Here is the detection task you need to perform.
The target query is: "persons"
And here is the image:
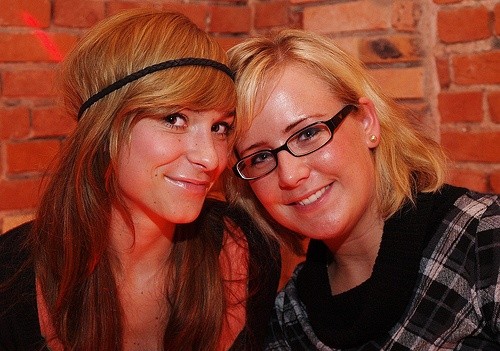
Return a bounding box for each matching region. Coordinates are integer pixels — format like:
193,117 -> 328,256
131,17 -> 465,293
0,8 -> 284,351
220,29 -> 500,351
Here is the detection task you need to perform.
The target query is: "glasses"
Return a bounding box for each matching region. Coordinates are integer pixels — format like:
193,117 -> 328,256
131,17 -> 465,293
232,104 -> 354,182
